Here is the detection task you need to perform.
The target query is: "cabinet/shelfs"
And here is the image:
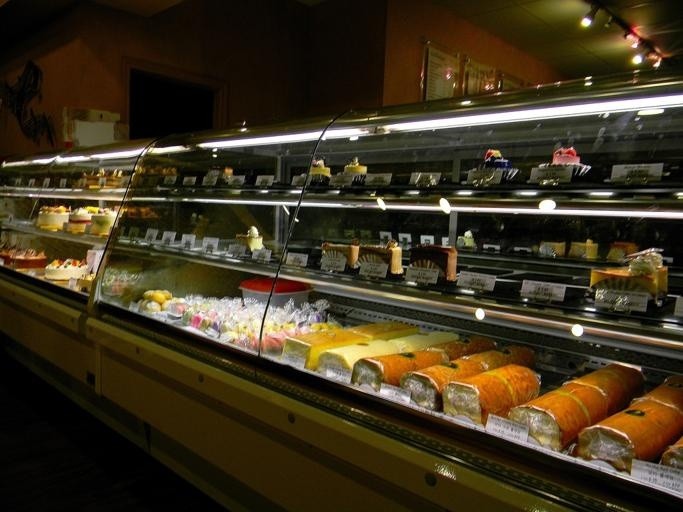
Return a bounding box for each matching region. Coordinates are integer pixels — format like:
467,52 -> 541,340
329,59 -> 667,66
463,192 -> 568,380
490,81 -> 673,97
0,139 -> 158,396
86,74 -> 683,511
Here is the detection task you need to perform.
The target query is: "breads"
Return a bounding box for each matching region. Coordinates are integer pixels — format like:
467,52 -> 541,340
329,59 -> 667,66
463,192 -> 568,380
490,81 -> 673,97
283,321 -> 418,371
137,288 -> 177,312
316,329 -> 461,380
399,345 -> 537,411
441,364 -> 542,426
660,436 -> 683,469
571,375 -> 681,472
507,363 -> 648,453
349,335 -> 498,392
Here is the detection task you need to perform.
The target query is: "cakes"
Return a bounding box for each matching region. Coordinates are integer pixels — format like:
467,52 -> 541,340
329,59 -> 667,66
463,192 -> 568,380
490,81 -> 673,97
357,239 -> 403,274
43,258 -> 88,280
35,206 -> 70,230
589,252 -> 668,295
5,247 -> 47,269
409,244 -> 457,281
568,239 -> 597,258
538,240 -> 566,257
309,158 -> 330,177
319,238 -> 360,268
343,157 -> 367,175
91,212 -> 116,234
62,206 -> 104,234
236,225 -> 265,251
551,146 -> 579,164
607,242 -> 632,259
74,169 -> 125,190
478,148 -> 510,169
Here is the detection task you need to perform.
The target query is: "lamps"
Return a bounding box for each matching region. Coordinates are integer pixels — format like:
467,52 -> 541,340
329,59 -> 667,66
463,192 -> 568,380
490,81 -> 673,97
577,3 -> 679,72
